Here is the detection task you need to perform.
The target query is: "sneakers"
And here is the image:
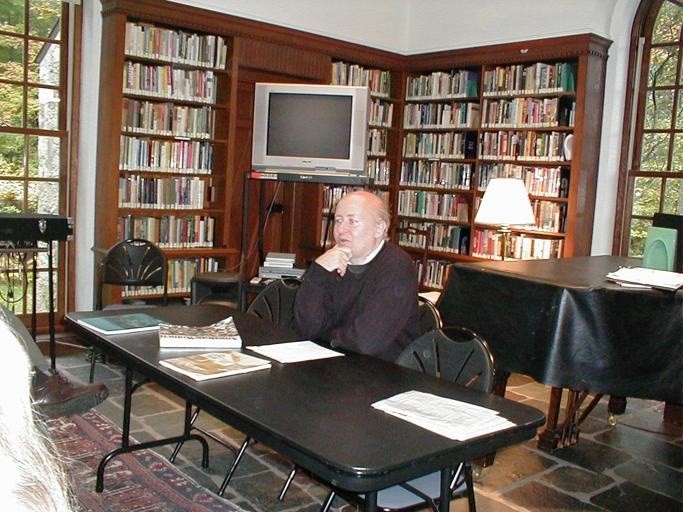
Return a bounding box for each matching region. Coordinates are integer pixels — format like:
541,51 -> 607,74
29,368 -> 109,420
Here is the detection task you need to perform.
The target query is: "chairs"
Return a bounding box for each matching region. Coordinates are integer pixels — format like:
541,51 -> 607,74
91,238 -> 168,383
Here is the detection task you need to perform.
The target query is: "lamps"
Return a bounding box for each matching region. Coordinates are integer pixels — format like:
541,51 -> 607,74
475,179 -> 538,261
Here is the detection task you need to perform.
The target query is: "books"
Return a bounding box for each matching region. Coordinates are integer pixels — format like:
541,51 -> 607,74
614,281 -> 652,288
77,313 -> 170,335
265,251 -> 297,264
158,347 -> 272,382
399,72 -> 478,288
321,51 -> 390,246
473,62 -> 576,259
605,265 -> 683,292
263,262 -> 294,268
158,315 -> 243,350
122,18 -> 228,298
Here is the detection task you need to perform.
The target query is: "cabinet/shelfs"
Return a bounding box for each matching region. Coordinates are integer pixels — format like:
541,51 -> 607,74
91,1 -> 612,363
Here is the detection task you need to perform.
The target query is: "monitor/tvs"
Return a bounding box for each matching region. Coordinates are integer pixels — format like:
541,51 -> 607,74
251,82 -> 371,176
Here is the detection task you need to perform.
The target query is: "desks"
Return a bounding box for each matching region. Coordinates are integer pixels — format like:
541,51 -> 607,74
450,254 -> 681,468
60,303 -> 545,512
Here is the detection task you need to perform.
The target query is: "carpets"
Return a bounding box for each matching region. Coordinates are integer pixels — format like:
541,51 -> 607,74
37,408 -> 251,512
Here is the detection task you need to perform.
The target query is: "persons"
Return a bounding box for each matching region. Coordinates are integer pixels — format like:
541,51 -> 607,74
0,303 -> 108,421
0,319 -> 77,512
290,189 -> 422,364
